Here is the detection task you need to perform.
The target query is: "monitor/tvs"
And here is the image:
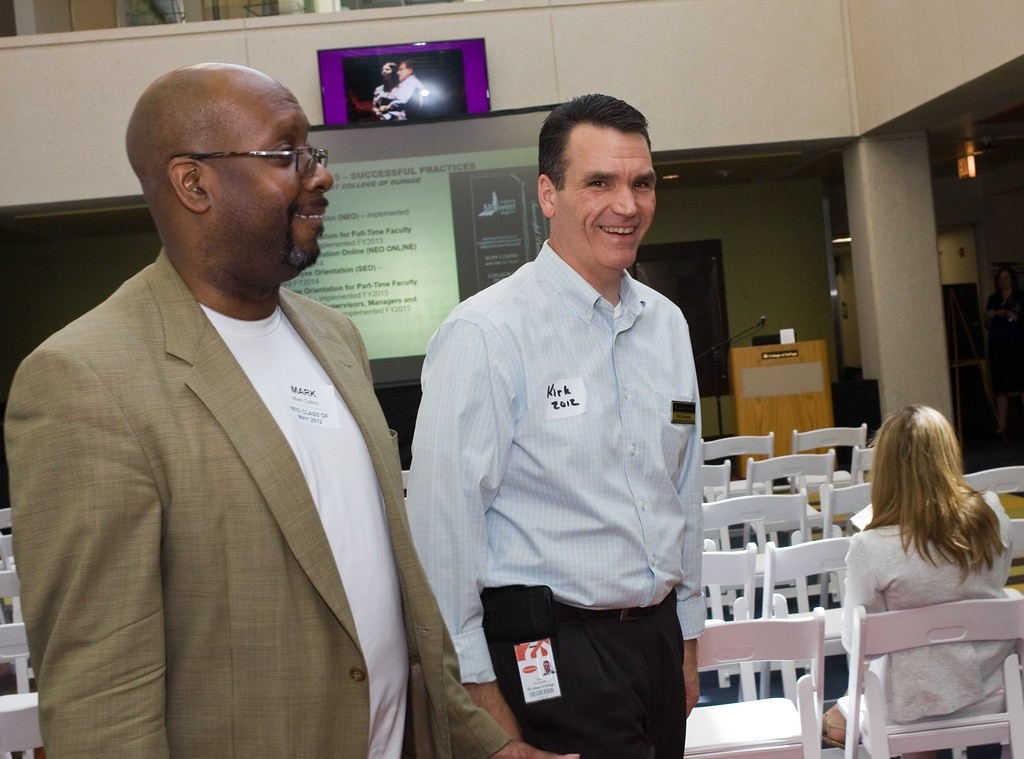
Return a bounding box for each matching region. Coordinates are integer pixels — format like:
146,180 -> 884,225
317,36 -> 491,126
751,333 -> 797,348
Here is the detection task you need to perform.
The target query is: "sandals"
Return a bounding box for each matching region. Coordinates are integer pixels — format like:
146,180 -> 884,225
821,712 -> 846,751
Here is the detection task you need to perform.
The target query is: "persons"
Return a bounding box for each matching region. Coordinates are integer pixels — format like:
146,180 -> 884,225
985,266 -> 1024,434
543,660 -> 555,676
4,63 -> 578,759
372,61 -> 425,120
408,95 -> 705,759
822,405 -> 1014,759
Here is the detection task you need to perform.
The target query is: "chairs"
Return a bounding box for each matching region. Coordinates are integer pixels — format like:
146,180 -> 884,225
0,421 -> 1024,759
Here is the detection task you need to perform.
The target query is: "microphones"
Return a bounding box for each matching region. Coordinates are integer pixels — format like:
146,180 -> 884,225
761,316 -> 767,327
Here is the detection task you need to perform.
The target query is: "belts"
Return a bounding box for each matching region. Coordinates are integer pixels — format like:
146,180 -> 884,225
555,587 -> 675,626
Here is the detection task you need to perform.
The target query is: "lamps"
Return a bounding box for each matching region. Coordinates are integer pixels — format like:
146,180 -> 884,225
957,154 -> 976,178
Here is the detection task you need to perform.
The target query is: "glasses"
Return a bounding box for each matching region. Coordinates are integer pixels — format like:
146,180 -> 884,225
170,146 -> 328,178
